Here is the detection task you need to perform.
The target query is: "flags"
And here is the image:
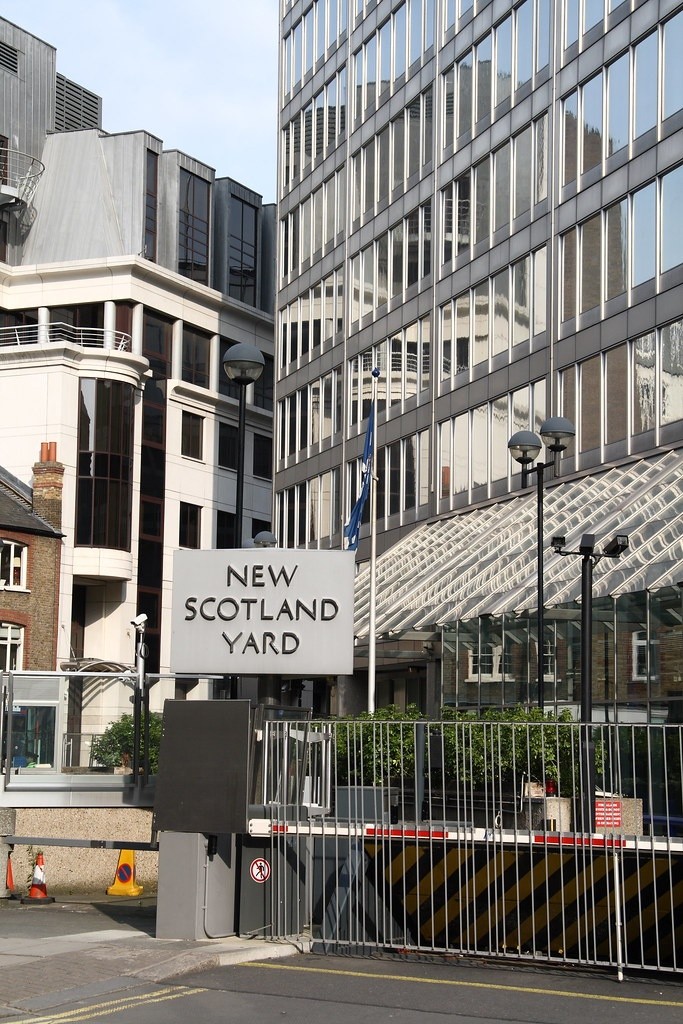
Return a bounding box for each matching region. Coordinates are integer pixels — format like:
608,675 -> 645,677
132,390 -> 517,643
343,381 -> 374,556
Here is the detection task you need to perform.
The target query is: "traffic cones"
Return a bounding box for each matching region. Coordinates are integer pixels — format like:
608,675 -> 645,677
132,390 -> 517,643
107,848 -> 144,897
21,851 -> 56,904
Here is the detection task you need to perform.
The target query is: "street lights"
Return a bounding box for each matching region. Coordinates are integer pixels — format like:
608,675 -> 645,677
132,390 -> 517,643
507,417 -> 576,720
224,344 -> 265,699
552,533 -> 629,834
